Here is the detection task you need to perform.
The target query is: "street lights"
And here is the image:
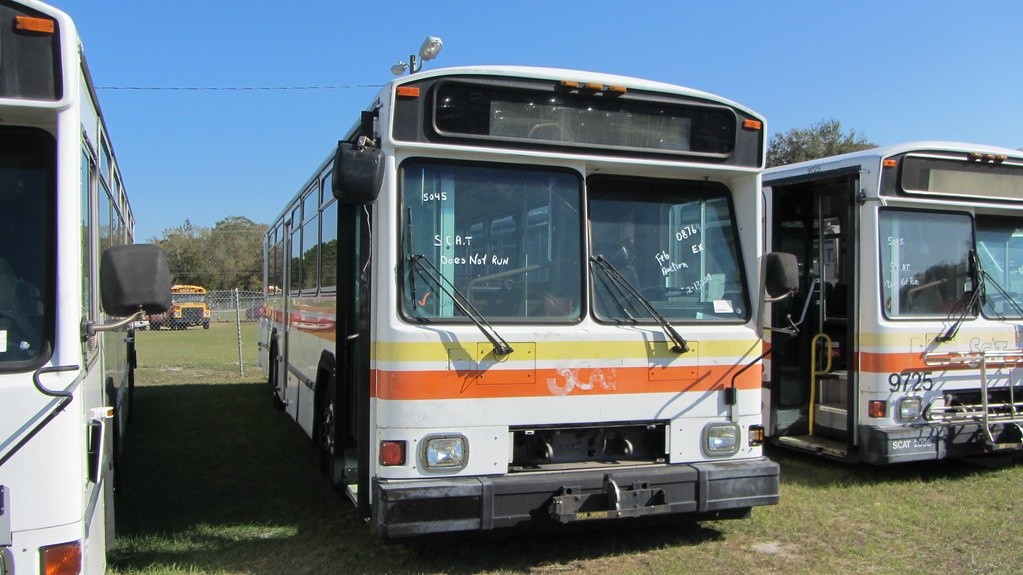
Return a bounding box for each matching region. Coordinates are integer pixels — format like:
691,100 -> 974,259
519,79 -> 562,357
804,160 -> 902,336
390,35 -> 443,79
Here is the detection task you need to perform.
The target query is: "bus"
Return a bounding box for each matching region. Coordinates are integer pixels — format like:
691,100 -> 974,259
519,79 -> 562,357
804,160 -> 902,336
148,285 -> 211,330
256,63 -> 800,547
0,0 -> 177,575
466,139 -> 1023,465
